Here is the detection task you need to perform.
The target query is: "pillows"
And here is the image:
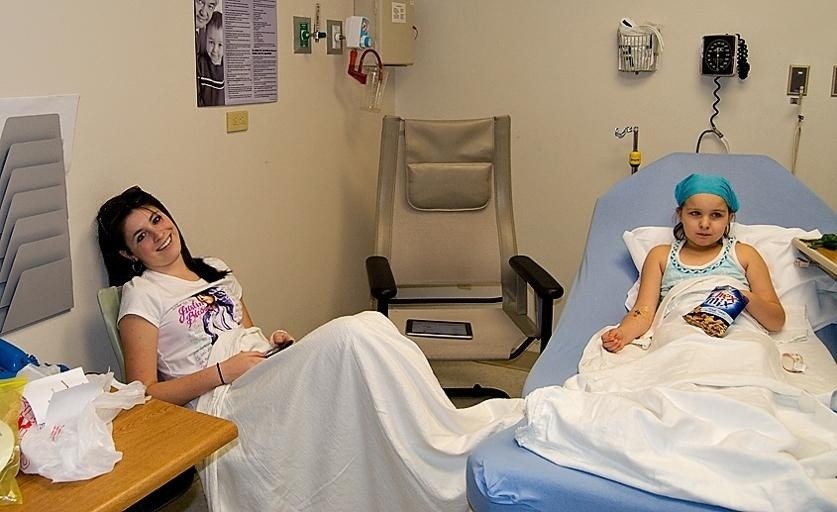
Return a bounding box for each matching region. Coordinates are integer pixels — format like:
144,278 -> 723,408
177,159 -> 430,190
621,217 -> 837,335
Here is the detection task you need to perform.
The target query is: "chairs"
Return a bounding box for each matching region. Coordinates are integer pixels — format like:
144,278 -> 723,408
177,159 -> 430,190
95,279 -> 138,384
361,110 -> 564,404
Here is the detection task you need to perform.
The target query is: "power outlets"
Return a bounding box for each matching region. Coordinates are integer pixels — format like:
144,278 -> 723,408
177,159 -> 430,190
224,108 -> 249,134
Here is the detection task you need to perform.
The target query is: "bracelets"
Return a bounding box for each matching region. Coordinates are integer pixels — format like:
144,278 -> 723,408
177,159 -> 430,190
214,360 -> 228,387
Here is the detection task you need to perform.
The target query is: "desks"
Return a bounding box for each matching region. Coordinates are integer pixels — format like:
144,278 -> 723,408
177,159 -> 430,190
0,386 -> 240,511
789,223 -> 835,279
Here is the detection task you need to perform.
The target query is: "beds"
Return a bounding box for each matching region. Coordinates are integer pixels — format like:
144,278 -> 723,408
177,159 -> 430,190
464,149 -> 836,509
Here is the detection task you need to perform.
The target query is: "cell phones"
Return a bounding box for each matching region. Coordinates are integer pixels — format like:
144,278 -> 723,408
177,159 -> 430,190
262,340 -> 293,358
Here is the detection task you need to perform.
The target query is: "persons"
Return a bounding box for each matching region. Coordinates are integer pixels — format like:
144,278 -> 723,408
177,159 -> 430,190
94,185 -> 296,408
196,11 -> 224,105
194,0 -> 218,55
600,174 -> 787,352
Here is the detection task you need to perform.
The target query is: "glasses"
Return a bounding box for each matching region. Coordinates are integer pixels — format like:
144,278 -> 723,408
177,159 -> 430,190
96,185 -> 141,229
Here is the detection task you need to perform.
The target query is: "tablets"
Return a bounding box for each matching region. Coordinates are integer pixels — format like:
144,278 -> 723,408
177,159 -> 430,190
406,318 -> 473,340
